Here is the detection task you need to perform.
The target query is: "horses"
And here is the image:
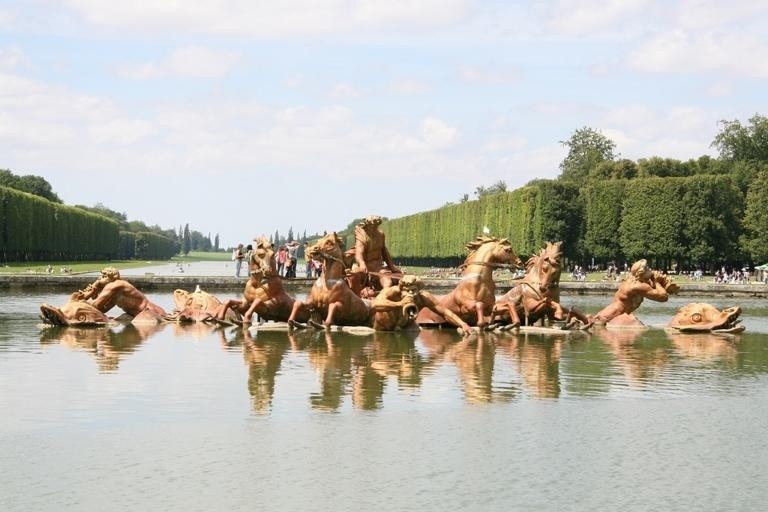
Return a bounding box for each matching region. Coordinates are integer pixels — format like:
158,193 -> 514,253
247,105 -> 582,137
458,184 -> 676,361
217,233 -> 307,330
287,230 -> 373,330
414,235 -> 525,336
493,240 -> 589,330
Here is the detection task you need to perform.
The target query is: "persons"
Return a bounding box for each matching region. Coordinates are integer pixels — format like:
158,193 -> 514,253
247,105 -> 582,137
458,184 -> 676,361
572,264 -> 621,282
83,267 -> 166,319
353,214 -> 402,289
235,241 -> 323,279
713,267 -> 753,284
595,259 -> 668,322
513,269 -> 524,280
